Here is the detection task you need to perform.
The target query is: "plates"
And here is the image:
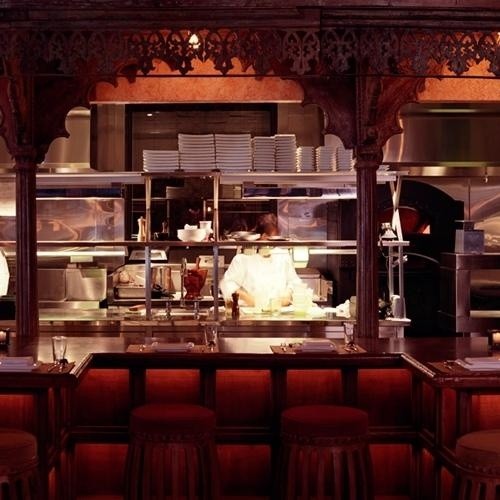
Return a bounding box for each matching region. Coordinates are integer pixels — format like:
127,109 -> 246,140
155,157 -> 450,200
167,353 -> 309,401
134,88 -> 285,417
167,185 -> 189,201
223,186 -> 242,199
233,230 -> 261,240
455,354 -> 500,373
142,132 -> 355,174
375,163 -> 391,171
301,339 -> 337,353
0,357 -> 41,373
151,342 -> 193,353
268,235 -> 290,241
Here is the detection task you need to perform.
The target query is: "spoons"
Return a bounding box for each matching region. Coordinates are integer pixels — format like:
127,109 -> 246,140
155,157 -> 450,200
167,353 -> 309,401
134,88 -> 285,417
47,359 -> 68,373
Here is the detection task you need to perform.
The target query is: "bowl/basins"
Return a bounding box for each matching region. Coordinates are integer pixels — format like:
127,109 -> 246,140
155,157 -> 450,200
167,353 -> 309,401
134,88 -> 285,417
176,229 -> 206,241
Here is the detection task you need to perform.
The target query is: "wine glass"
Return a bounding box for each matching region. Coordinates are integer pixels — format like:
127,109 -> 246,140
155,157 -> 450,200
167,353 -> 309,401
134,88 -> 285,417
182,268 -> 208,299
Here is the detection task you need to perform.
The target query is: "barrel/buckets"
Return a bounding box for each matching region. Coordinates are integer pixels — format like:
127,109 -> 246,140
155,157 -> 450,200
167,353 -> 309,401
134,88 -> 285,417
81,275 -> 106,301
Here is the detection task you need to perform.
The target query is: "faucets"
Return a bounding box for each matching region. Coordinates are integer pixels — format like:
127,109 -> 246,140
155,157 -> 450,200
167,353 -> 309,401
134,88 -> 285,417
180,259 -> 189,306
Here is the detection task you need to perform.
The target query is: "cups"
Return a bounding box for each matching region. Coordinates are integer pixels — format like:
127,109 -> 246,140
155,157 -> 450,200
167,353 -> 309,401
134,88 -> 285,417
200,221 -> 211,240
344,326 -> 354,349
204,323 -> 218,349
52,336 -> 67,367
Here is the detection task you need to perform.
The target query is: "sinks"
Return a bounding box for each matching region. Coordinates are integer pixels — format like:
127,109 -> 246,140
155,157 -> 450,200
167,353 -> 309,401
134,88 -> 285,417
156,311 -> 208,315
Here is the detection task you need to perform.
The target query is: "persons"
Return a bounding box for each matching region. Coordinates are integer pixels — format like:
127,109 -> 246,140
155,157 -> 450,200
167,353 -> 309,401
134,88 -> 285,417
220,213 -> 314,307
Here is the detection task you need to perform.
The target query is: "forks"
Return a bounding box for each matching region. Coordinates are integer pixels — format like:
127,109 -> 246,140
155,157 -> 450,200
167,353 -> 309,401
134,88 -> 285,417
281,343 -> 286,352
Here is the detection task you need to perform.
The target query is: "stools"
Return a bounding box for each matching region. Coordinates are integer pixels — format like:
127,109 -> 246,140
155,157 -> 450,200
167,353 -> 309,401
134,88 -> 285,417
452,429 -> 500,498
275,406 -> 373,500
123,402 -> 219,499
0,427 -> 43,498
